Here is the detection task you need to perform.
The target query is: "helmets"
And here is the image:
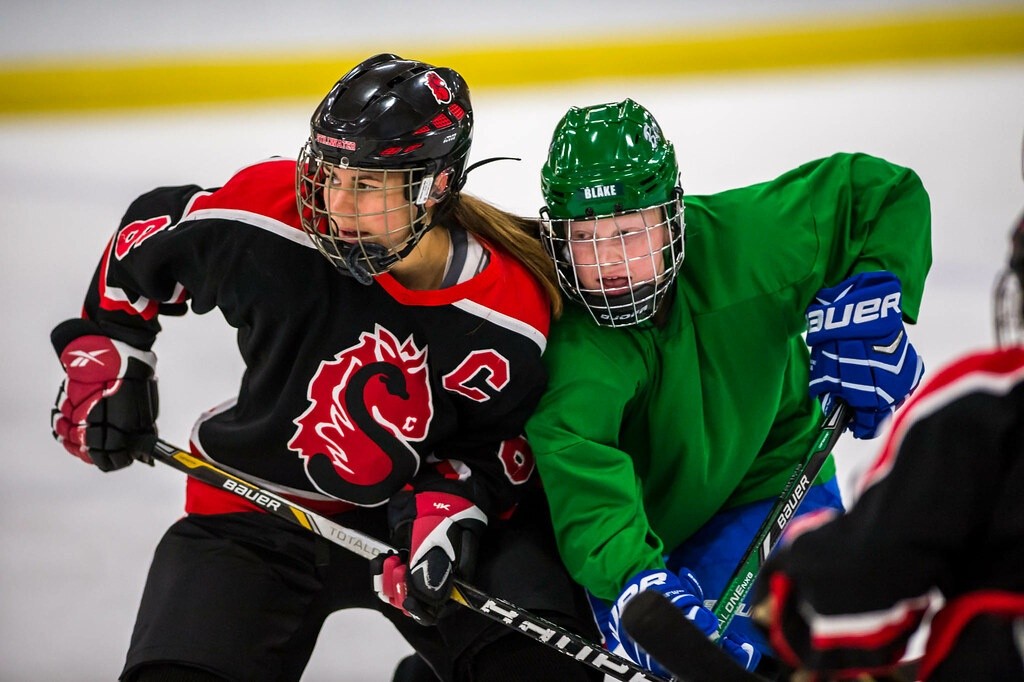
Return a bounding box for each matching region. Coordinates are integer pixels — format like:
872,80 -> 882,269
294,54 -> 474,284
539,98 -> 686,327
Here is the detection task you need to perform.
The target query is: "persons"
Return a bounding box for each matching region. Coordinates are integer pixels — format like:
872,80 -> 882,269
523,99 -> 933,682
45,53 -> 604,682
766,212 -> 1024,682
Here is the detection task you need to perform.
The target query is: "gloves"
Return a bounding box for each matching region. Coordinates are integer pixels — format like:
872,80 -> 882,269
608,566 -> 761,682
804,271 -> 925,440
50,318 -> 158,472
370,459 -> 520,627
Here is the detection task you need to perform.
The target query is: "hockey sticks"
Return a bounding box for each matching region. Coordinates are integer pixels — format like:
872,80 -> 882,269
152,437 -> 671,682
668,396 -> 851,682
